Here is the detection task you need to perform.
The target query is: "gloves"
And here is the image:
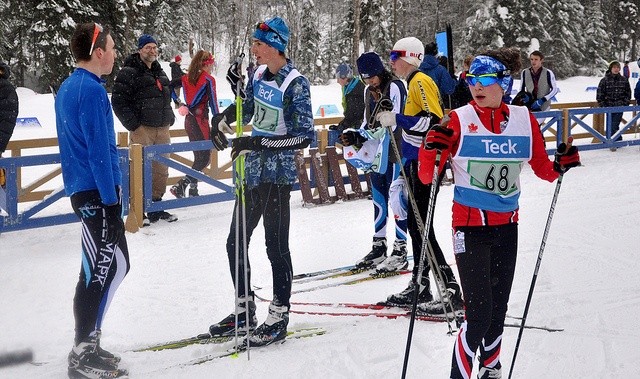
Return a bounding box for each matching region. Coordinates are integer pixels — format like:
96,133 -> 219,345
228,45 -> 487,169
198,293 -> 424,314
553,143 -> 580,176
231,136 -> 262,162
337,119 -> 348,133
521,93 -> 529,103
338,131 -> 360,146
424,124 -> 454,151
211,114 -> 234,151
531,97 -> 547,109
104,204 -> 126,246
376,112 -> 396,127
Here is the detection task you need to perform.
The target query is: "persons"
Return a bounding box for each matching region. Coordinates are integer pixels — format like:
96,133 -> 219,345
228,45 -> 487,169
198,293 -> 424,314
111,34 -> 179,226
55,21 -> 133,379
623,61 -> 630,80
337,52 -> 411,274
373,37 -> 465,319
0,62 -> 19,154
170,49 -> 219,199
334,62 -> 366,131
633,57 -> 640,106
417,49 -> 581,379
460,54 -> 476,83
169,56 -> 185,108
420,42 -> 458,95
521,51 -> 559,150
596,61 -> 631,142
208,17 -> 316,346
437,55 -> 448,69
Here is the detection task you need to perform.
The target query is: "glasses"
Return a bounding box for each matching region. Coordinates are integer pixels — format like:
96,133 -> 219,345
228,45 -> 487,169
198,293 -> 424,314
390,50 -> 424,62
256,22 -> 286,43
466,70 -> 511,87
89,23 -> 103,56
140,46 -> 158,52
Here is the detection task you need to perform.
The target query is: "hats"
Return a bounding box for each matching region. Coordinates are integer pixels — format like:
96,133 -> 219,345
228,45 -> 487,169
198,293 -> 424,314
390,37 -> 424,68
425,42 -> 438,56
175,55 -> 181,62
356,52 -> 385,79
138,34 -> 158,50
336,64 -> 354,79
252,17 -> 289,52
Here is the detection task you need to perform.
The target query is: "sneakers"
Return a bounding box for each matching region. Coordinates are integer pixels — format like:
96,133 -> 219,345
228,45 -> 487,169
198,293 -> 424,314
147,211 -> 178,223
375,238 -> 409,273
143,212 -> 150,226
68,330 -> 128,378
170,178 -> 191,199
209,291 -> 258,337
189,182 -> 199,196
387,263 -> 433,305
95,332 -> 121,364
243,301 -> 290,347
418,265 -> 464,315
355,237 -> 387,267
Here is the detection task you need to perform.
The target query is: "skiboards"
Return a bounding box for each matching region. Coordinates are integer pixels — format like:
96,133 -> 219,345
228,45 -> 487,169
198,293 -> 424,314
288,303 -> 564,332
254,255 -> 414,301
129,326 -> 326,373
